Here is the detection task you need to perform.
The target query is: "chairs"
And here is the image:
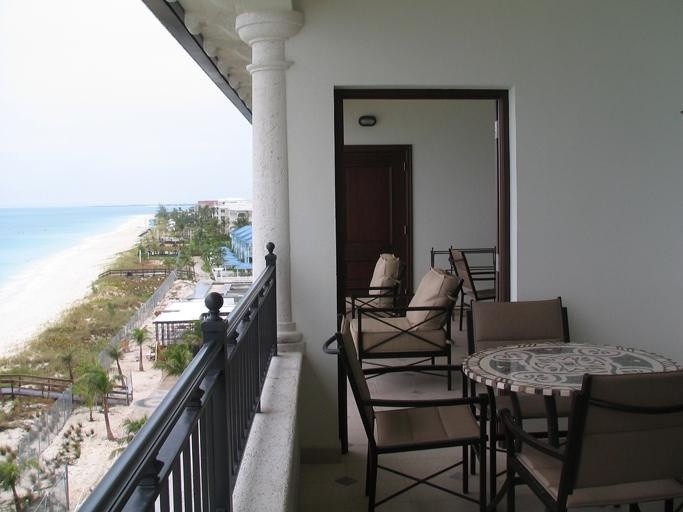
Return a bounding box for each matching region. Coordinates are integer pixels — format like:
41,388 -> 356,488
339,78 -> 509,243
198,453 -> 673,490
322,312 -> 488,511
449,249 -> 496,332
344,260 -> 403,319
498,369 -> 683,511
351,265 -> 464,391
466,296 -> 571,475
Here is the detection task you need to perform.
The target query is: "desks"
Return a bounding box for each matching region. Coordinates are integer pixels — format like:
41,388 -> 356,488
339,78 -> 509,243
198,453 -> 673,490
462,341 -> 682,512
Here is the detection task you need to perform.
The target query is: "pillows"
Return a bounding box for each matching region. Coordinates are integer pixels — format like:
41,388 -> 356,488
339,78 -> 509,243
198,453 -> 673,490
406,267 -> 462,331
368,253 -> 400,305
